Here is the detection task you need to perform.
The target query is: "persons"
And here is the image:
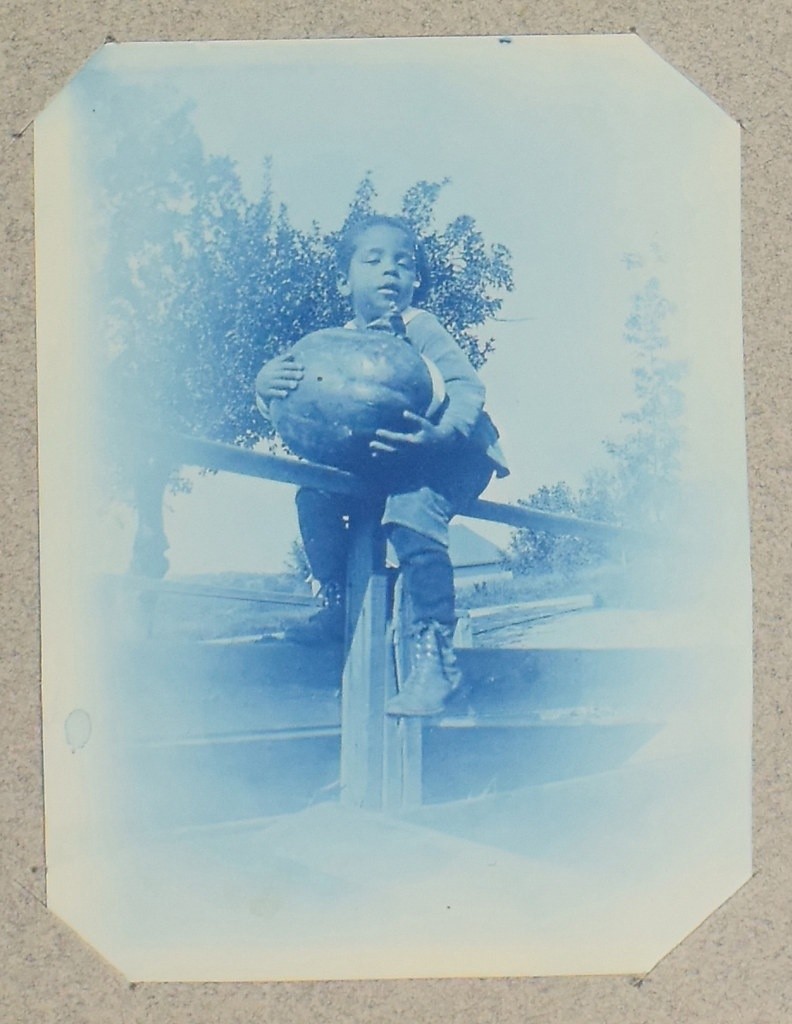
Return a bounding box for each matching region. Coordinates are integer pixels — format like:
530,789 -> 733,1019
250,212 -> 514,722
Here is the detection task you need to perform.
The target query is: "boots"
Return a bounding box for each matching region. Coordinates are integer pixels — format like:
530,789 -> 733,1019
281,577 -> 345,645
384,620 -> 467,718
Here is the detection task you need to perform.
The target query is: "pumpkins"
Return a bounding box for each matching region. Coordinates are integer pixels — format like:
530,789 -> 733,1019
266,326 -> 448,471
